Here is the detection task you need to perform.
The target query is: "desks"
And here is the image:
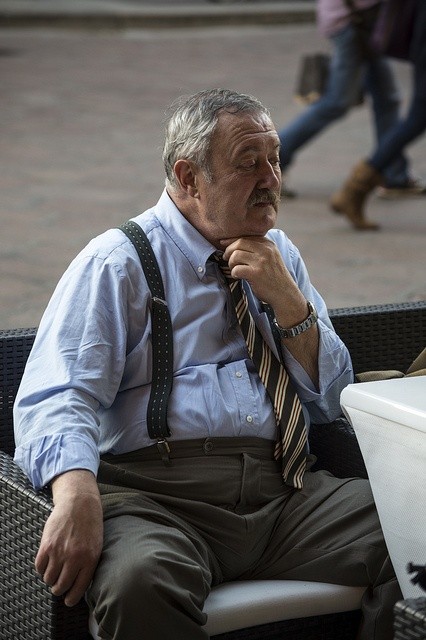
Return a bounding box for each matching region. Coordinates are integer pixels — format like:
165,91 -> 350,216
339,374 -> 426,601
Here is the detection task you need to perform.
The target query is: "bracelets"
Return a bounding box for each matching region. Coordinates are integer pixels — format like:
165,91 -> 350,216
273,300 -> 318,338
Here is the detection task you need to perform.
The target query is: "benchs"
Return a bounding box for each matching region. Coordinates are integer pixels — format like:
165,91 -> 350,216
0,299 -> 426,640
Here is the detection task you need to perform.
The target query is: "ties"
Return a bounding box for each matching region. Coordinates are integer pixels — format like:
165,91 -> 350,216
209,250 -> 307,489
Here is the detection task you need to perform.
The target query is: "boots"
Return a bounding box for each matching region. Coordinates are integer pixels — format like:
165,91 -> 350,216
329,160 -> 380,229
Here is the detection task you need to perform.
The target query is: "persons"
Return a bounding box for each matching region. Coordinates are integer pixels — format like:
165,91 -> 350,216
273,1 -> 426,203
327,1 -> 426,231
9,85 -> 406,637
